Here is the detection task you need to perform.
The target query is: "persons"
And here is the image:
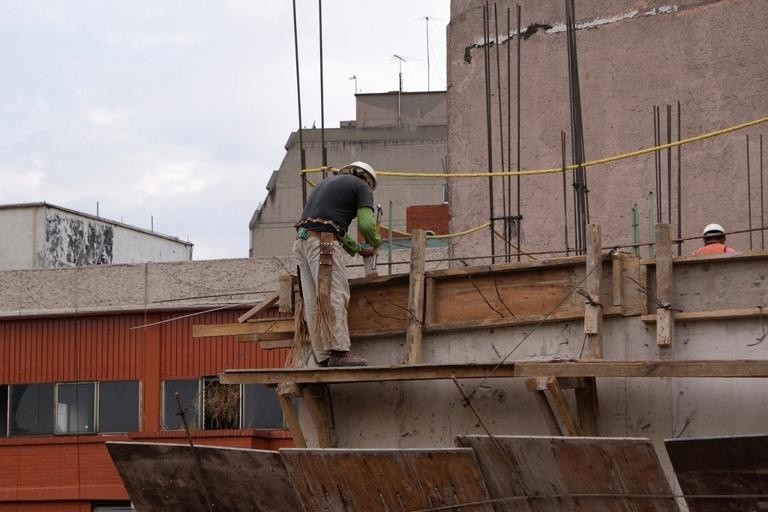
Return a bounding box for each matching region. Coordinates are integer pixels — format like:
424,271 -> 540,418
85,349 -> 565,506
691,222 -> 736,256
292,161 -> 382,367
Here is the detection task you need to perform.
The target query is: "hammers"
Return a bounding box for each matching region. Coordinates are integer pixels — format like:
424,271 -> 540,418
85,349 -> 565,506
371,204 -> 383,270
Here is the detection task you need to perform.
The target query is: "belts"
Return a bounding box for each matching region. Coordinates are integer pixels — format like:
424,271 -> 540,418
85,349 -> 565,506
295,228 -> 342,243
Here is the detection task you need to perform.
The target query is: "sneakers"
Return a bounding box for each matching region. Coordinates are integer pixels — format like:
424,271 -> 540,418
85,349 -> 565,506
328,352 -> 369,367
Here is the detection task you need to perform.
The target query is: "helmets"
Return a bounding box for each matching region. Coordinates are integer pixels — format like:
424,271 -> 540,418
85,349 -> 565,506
701,223 -> 727,242
337,159 -> 379,192
